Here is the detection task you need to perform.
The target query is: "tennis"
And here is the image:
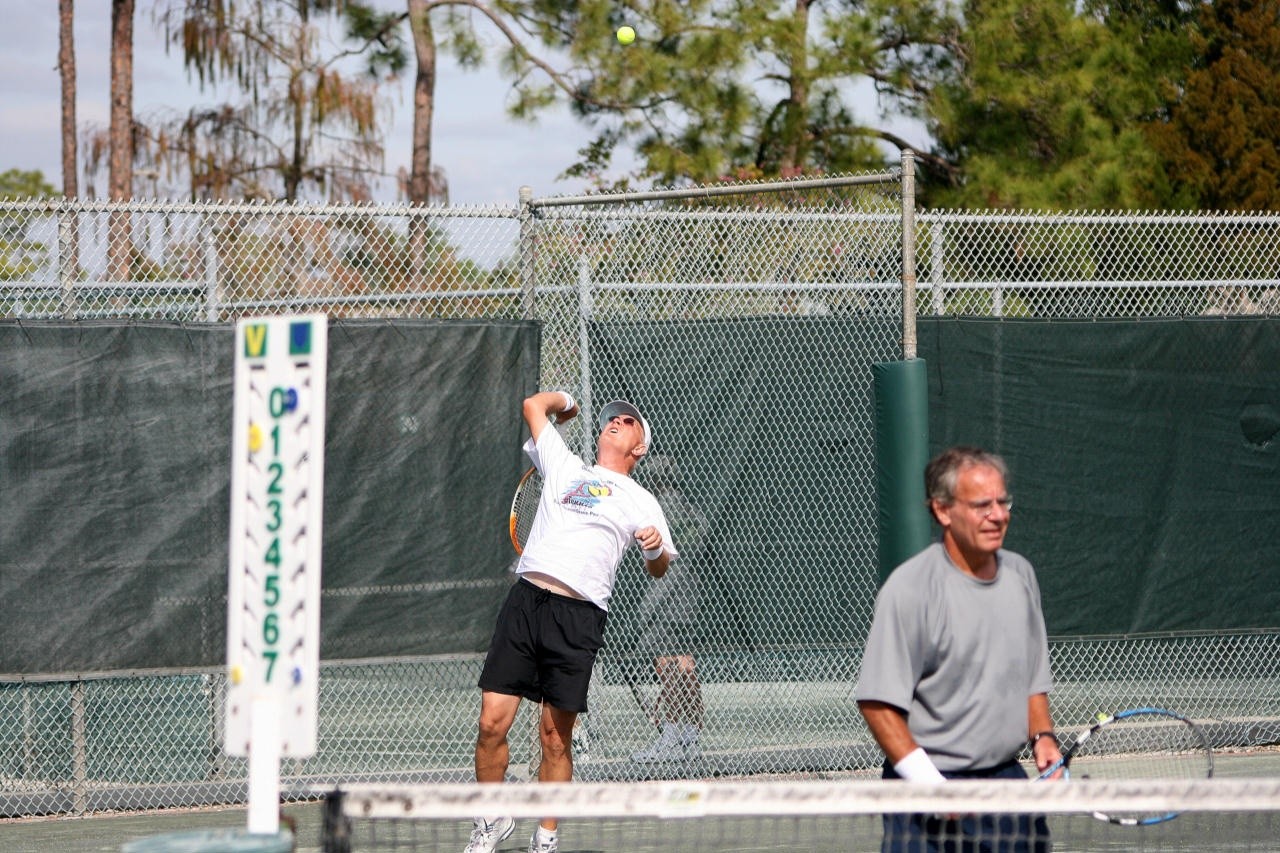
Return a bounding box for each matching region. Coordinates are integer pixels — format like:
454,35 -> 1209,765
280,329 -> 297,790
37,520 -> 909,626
617,26 -> 636,45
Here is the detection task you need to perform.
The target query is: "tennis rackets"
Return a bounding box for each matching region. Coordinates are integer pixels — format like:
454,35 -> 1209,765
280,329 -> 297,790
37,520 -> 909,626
509,416 -> 558,556
934,708 -> 1214,826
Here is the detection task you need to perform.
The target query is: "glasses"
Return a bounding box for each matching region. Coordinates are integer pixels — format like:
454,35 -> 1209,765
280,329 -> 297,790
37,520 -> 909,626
951,494 -> 1013,517
608,415 -> 643,442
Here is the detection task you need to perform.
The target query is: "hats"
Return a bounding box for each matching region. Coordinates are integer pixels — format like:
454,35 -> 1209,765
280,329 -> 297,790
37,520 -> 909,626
599,400 -> 651,461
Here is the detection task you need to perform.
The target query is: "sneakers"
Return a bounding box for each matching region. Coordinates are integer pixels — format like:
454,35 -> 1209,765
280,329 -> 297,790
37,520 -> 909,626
463,815 -> 516,853
527,828 -> 558,853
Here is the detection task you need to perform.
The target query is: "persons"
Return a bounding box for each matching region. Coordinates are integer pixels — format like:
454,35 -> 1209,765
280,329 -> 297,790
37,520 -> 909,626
853,446 -> 1067,853
460,392 -> 680,853
569,656 -> 604,755
622,447 -> 704,764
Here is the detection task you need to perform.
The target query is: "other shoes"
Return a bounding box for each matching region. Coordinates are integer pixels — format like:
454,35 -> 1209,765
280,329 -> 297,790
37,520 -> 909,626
629,740 -> 686,763
570,730 -> 589,761
683,741 -> 701,762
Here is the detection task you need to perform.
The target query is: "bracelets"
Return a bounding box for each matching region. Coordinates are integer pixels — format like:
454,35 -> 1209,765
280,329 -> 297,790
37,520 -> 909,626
641,542 -> 665,562
1030,732 -> 1060,750
558,390 -> 574,412
